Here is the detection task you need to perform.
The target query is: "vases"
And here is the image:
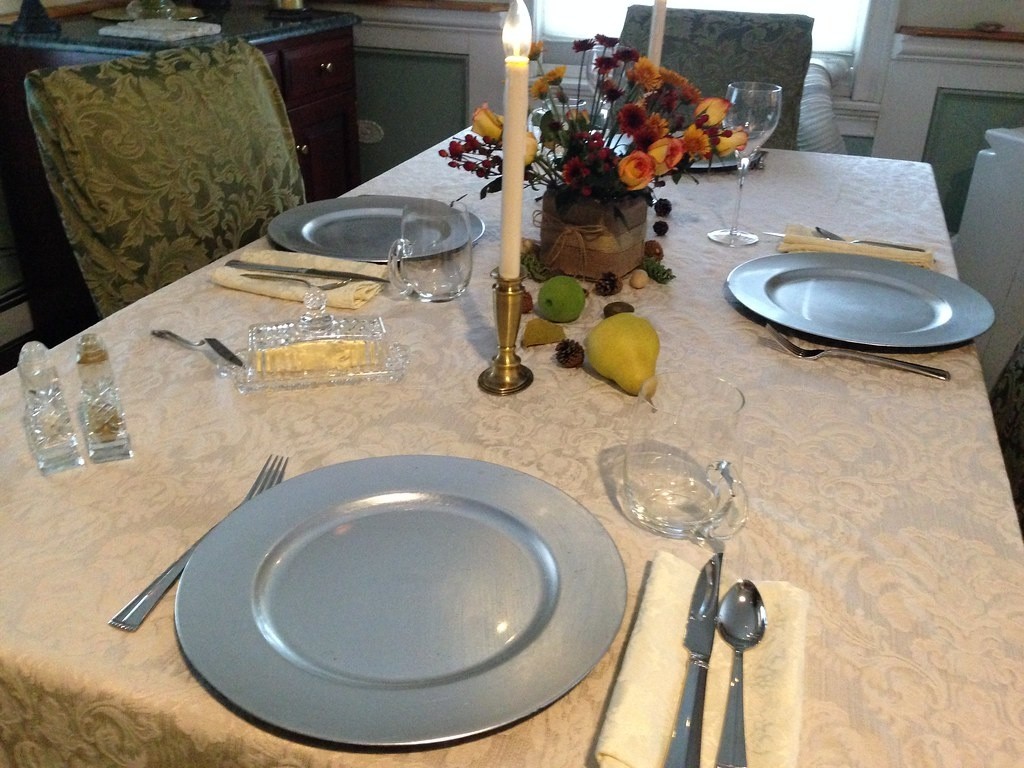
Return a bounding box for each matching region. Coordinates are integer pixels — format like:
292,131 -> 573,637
539,188 -> 649,279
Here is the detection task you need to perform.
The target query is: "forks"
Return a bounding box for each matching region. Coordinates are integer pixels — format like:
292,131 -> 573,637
765,323 -> 953,381
105,454 -> 290,631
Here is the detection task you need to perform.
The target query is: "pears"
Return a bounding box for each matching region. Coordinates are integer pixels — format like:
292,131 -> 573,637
585,313 -> 660,412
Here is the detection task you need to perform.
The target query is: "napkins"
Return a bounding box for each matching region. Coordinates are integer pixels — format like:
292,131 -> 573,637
592,548 -> 812,768
210,247 -> 393,308
777,222 -> 936,271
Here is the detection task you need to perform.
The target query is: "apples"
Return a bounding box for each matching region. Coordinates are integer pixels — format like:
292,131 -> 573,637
537,275 -> 585,323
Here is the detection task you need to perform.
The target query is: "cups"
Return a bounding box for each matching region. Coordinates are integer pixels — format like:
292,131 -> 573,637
388,201 -> 473,301
618,372 -> 752,539
528,98 -> 587,159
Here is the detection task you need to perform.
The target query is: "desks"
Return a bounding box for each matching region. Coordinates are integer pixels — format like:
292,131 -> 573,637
0,120 -> 1024,768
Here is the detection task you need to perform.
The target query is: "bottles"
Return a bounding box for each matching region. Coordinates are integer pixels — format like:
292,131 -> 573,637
77,334 -> 131,462
14,340 -> 84,473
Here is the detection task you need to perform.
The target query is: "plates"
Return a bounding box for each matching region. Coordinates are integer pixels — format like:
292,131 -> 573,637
600,138 -> 762,170
268,196 -> 484,262
726,253 -> 995,349
172,453 -> 628,746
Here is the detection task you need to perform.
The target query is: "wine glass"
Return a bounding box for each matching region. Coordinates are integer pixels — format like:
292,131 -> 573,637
586,47 -> 630,137
707,81 -> 782,247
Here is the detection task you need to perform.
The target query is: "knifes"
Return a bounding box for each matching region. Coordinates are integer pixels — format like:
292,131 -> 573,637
765,232 -> 832,240
666,552 -> 723,768
226,259 -> 390,285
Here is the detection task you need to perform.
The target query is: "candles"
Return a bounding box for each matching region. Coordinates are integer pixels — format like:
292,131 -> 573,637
645,1 -> 667,98
499,0 -> 533,278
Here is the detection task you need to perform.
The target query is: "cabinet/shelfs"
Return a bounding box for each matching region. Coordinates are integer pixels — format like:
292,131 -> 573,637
0,4 -> 364,345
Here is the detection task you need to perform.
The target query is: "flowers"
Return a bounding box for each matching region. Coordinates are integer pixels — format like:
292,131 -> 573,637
438,31 -> 749,236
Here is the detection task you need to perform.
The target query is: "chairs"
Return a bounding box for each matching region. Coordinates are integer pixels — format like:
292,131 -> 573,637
610,4 -> 816,149
24,35 -> 310,315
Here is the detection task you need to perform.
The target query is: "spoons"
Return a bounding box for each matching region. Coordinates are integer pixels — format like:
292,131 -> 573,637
712,579 -> 765,768
814,227 -> 926,251
243,273 -> 355,289
